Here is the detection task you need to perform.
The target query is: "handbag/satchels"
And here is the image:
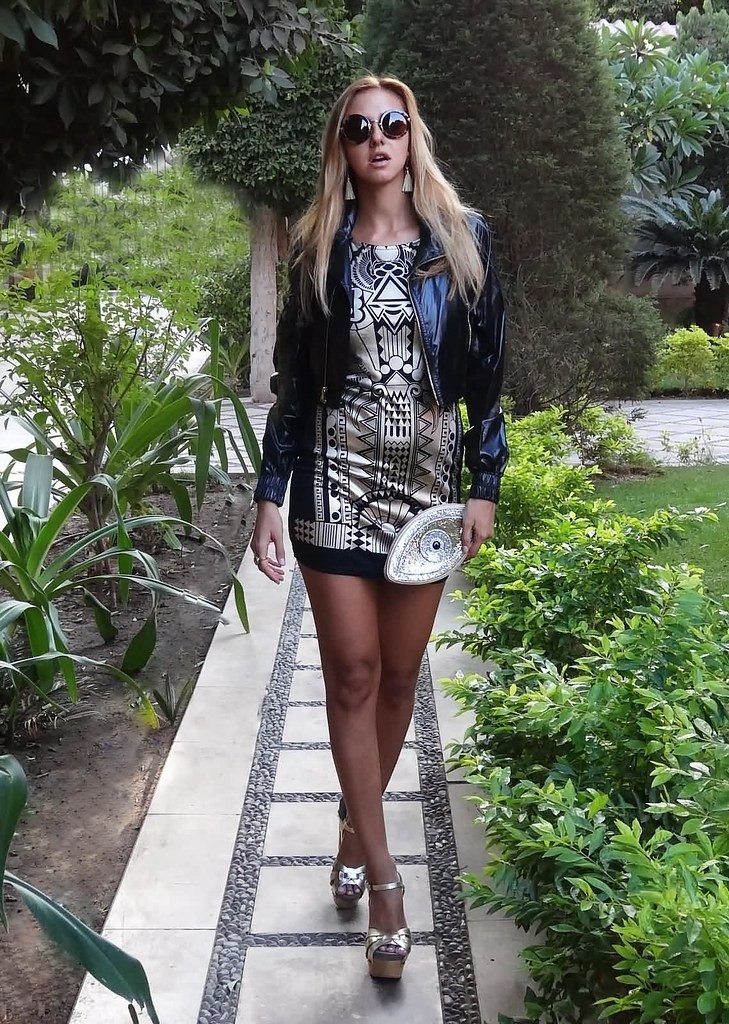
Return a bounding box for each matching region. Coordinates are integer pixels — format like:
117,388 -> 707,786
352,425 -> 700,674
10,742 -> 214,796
383,503 -> 475,585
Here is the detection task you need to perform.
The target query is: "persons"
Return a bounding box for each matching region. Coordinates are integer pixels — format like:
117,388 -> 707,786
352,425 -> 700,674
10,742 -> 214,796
251,72 -> 510,982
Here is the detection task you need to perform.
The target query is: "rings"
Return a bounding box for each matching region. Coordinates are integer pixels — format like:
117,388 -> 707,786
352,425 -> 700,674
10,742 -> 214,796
254,555 -> 268,565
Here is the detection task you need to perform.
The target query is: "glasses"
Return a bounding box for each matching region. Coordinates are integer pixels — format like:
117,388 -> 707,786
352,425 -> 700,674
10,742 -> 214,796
339,109 -> 412,143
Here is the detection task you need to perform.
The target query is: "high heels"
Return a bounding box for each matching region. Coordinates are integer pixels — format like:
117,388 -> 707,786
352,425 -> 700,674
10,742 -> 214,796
329,795 -> 370,908
365,868 -> 413,981
